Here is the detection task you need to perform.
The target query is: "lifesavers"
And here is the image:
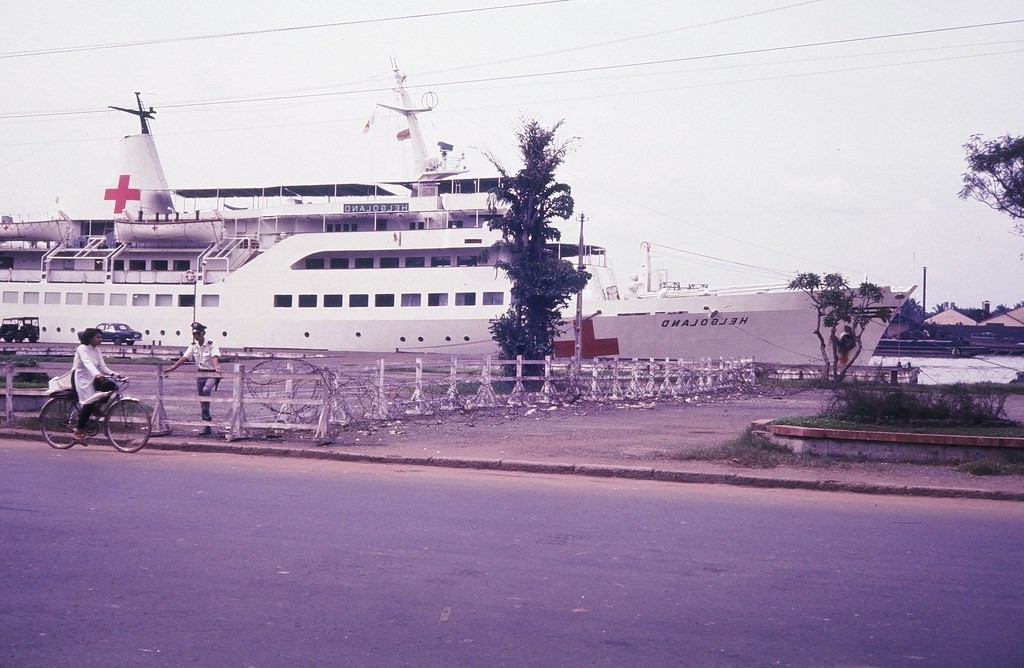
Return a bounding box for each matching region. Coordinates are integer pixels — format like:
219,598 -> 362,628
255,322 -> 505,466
185,270 -> 195,281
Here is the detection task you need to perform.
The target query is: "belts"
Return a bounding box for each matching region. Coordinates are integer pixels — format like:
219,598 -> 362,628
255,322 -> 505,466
198,369 -> 215,372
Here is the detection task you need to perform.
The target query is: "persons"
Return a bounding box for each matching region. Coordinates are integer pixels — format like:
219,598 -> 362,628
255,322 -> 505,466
163,323 -> 221,435
68,327 -> 125,447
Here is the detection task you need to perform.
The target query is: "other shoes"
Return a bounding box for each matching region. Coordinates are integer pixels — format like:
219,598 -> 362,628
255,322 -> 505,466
68,418 -> 77,424
199,427 -> 211,435
70,433 -> 87,446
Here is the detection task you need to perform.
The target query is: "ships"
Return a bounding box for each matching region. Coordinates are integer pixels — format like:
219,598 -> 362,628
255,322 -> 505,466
5,58 -> 916,365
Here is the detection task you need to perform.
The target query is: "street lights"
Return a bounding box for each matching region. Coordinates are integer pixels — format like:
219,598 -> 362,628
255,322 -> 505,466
923,264 -> 932,323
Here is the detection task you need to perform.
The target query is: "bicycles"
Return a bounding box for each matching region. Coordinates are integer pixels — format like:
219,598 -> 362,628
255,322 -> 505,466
39,372 -> 152,454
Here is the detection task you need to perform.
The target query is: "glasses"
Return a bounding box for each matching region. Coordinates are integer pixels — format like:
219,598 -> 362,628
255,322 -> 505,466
193,332 -> 202,336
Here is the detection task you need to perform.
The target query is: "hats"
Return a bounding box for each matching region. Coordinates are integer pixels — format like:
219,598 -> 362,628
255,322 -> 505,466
191,322 -> 207,333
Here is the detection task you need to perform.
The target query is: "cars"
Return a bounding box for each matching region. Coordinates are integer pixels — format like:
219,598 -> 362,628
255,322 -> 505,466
77,322 -> 144,346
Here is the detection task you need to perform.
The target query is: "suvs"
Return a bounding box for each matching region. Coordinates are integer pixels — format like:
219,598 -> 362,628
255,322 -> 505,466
0,316 -> 41,342
